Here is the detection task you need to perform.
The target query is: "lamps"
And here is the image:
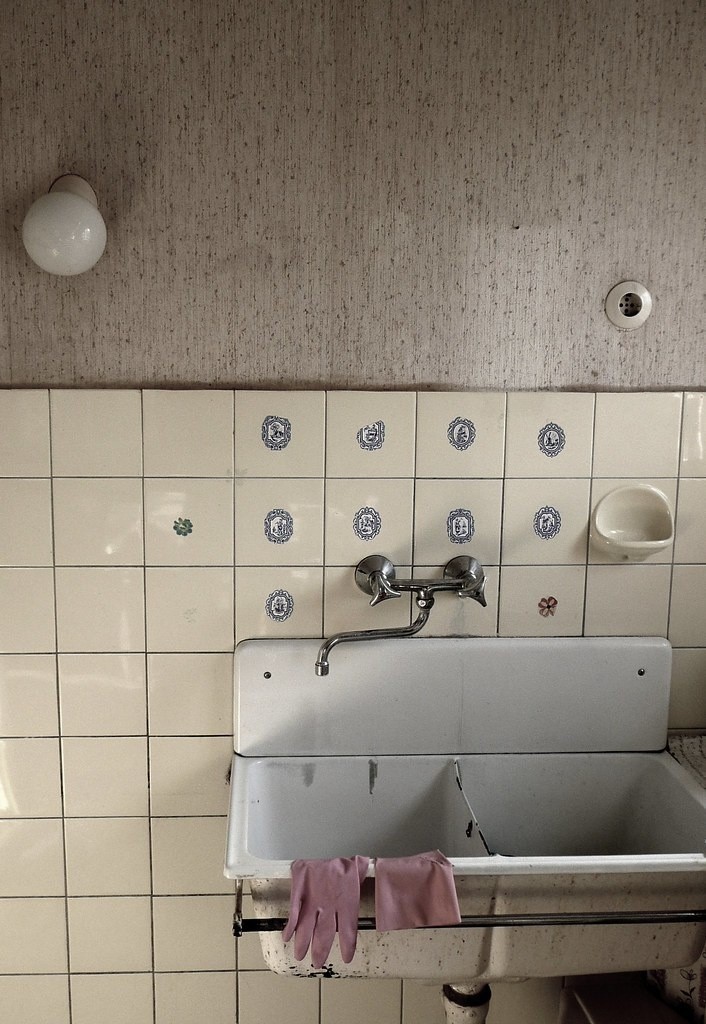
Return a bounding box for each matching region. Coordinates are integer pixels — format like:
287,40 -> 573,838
23,174 -> 110,277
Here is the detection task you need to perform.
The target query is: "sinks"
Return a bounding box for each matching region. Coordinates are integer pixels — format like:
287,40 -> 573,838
455,751 -> 706,979
223,754 -> 488,980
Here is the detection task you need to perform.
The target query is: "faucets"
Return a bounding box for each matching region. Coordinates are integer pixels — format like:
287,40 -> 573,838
315,555 -> 488,676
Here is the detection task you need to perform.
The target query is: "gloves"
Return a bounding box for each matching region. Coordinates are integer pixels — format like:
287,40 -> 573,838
281,855 -> 369,970
376,848 -> 462,931
